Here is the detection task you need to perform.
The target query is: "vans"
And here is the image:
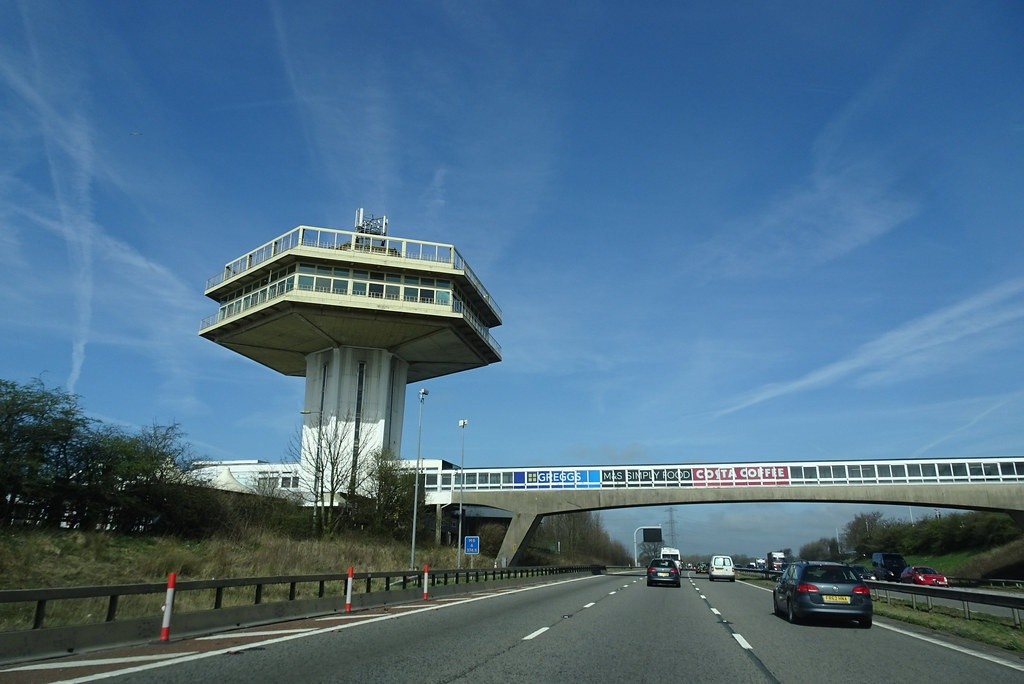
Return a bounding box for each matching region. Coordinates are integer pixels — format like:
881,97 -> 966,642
706,556 -> 736,582
872,552 -> 910,583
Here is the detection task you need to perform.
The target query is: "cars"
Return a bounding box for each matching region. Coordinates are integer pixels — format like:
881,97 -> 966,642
772,560 -> 873,629
900,566 -> 950,588
852,564 -> 877,581
778,563 -> 790,572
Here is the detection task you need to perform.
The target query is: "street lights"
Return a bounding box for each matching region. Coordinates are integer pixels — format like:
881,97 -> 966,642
457,419 -> 468,570
410,388 -> 430,574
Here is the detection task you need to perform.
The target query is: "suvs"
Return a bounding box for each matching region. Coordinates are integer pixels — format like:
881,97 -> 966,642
645,558 -> 680,588
685,561 -> 709,574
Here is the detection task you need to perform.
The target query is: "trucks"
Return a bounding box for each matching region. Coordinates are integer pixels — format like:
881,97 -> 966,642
768,551 -> 785,571
660,547 -> 684,575
747,558 -> 765,570
